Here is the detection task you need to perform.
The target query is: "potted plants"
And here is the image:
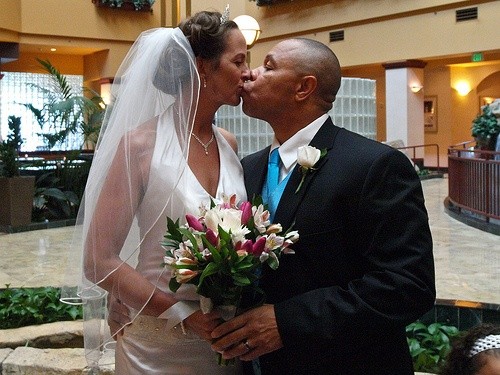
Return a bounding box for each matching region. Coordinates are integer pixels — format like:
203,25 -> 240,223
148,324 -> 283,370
0,57 -> 108,229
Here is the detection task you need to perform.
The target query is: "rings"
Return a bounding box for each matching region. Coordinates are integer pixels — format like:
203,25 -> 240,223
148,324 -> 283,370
244,340 -> 253,350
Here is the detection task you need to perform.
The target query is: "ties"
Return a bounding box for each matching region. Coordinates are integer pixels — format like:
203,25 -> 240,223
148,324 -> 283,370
267,148 -> 279,201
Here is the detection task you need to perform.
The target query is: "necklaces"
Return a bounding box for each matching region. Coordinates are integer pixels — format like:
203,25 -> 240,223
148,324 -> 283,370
173,103 -> 213,155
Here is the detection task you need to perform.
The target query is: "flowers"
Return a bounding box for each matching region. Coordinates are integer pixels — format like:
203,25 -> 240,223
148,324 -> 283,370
294,146 -> 328,193
159,194 -> 299,368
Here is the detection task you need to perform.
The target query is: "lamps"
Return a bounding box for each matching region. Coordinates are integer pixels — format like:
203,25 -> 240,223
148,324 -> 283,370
411,86 -> 423,93
234,15 -> 263,49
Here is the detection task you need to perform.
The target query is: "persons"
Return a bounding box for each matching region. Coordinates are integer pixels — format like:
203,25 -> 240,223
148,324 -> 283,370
58,10 -> 250,375
439,322 -> 500,375
107,38 -> 437,375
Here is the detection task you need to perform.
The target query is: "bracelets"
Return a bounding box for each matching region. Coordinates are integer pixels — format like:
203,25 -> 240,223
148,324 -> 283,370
180,318 -> 188,335
